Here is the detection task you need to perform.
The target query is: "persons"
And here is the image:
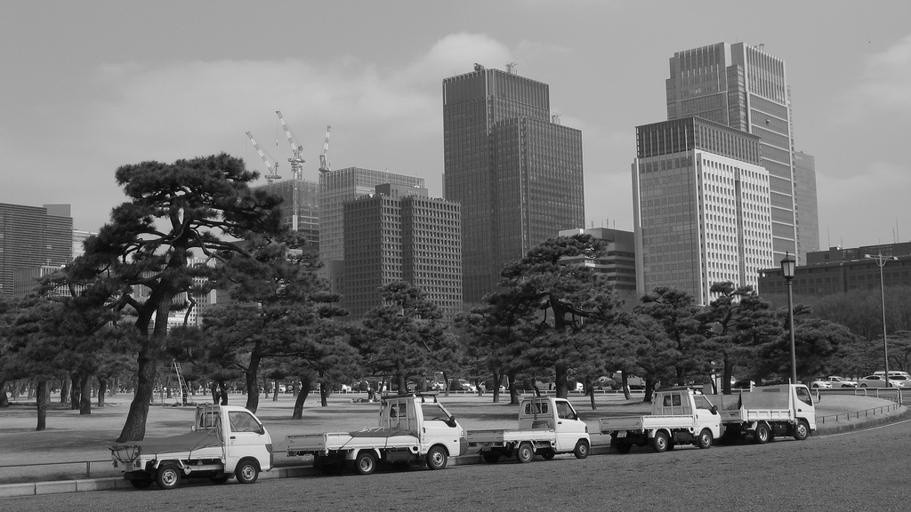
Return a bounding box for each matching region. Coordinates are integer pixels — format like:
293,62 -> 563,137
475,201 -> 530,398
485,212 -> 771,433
367,386 -> 378,402
220,388 -> 228,406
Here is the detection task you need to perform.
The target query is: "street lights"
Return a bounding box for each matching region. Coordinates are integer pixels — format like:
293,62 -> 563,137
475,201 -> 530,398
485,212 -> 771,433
862,250 -> 901,386
777,249 -> 801,382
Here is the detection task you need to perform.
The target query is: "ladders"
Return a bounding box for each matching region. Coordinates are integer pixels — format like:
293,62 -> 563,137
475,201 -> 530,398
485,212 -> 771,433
160,358 -> 193,406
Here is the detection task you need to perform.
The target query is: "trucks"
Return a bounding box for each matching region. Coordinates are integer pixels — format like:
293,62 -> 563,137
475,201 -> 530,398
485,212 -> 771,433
718,383 -> 828,444
596,389 -> 728,453
468,393 -> 598,465
285,390 -> 470,478
116,401 -> 271,486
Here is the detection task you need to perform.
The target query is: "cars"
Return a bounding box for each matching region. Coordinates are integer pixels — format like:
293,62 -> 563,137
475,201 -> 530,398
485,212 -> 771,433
812,368 -> 911,392
730,375 -> 757,388
